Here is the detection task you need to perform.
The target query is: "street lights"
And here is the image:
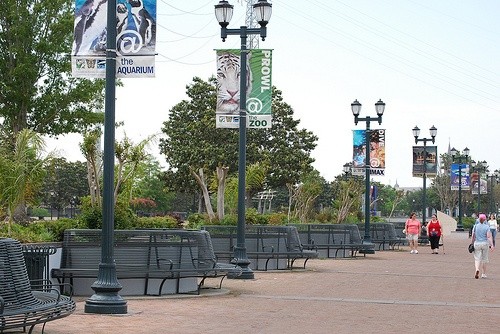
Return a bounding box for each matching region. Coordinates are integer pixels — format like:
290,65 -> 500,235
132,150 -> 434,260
213,0 -> 272,278
412,125 -> 438,243
350,98 -> 386,255
470,160 -> 488,219
486,169 -> 499,218
450,146 -> 470,232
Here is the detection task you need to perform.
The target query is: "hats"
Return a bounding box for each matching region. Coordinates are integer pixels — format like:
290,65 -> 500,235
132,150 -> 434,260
479,214 -> 486,220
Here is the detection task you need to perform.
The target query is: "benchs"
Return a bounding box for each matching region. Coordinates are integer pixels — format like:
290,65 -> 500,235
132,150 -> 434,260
0,222 -> 430,334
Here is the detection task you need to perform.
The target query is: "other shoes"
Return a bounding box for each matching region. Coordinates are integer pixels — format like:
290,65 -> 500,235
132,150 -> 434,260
475,269 -> 480,279
481,274 -> 487,279
410,249 -> 418,254
431,248 -> 438,254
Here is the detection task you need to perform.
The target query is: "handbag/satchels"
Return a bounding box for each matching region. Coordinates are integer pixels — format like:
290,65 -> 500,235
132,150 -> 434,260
429,231 -> 438,237
468,243 -> 474,253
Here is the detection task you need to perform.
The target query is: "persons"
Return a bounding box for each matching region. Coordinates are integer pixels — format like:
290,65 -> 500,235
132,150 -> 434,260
488,213 -> 498,248
426,214 -> 442,254
421,221 -> 434,245
469,213 -> 493,279
475,214 -> 488,226
405,213 -> 421,254
